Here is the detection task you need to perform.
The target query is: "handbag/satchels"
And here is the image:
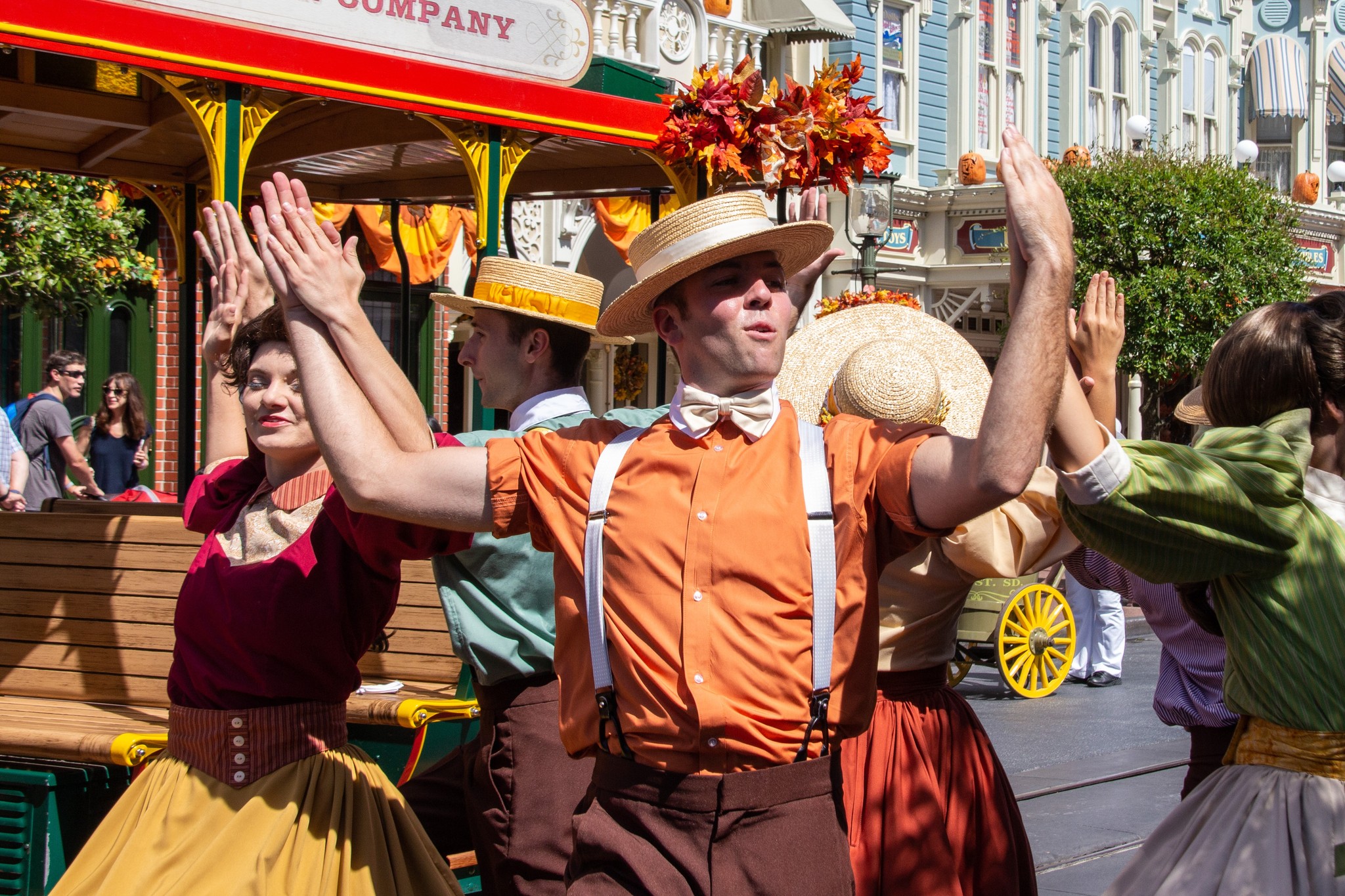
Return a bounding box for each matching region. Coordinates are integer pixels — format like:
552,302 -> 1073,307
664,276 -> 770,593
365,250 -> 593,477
108,484 -> 178,504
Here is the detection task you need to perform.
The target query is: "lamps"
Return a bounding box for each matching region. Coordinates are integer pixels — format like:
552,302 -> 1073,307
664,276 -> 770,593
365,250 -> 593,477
1327,160 -> 1345,192
1236,140 -> 1258,169
1127,115 -> 1150,152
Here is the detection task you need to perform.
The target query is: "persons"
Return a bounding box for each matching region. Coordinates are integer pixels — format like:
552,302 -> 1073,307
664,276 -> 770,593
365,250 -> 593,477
249,120 -> 1075,896
76,372 -> 154,493
777,162 -> 1125,896
19,349 -> 106,512
265,186 -> 845,896
1063,416 -> 1124,688
45,258 -> 475,896
1001,123 -> 1345,896
0,406 -> 30,512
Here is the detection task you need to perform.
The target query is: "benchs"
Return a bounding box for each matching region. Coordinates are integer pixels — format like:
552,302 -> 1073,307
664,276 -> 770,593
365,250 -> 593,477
0,496 -> 485,768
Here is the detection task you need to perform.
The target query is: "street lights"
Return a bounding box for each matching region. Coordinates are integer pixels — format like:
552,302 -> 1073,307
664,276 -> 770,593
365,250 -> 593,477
830,170 -> 907,297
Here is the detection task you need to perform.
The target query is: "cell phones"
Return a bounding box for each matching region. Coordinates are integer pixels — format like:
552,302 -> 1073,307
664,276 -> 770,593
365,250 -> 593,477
135,439 -> 145,460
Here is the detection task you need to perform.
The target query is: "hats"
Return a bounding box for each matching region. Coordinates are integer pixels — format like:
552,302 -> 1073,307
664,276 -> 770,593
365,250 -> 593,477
596,191 -> 835,337
429,256 -> 635,345
1174,337 -> 1222,427
774,303 -> 993,439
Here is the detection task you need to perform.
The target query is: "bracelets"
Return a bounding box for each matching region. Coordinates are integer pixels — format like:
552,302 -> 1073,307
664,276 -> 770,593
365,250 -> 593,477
0,489 -> 9,501
9,490 -> 23,495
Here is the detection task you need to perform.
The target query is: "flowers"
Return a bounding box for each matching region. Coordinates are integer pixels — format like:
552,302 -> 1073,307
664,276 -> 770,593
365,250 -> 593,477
814,289 -> 923,319
649,53 -> 896,198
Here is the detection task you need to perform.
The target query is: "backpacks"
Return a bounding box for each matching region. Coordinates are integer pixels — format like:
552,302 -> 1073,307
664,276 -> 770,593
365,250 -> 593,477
2,392 -> 63,462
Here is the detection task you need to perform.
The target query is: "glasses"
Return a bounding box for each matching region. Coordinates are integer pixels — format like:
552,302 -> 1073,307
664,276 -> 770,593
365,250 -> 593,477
101,386 -> 129,396
59,370 -> 87,379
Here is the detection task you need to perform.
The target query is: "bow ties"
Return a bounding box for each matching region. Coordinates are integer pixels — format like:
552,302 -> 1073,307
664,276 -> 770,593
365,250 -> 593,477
680,387 -> 775,439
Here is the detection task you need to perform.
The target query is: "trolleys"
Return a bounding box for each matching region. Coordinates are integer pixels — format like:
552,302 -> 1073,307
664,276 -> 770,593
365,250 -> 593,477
944,564 -> 1077,700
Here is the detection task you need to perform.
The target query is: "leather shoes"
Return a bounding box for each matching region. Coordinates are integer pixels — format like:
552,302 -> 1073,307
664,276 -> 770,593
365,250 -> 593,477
1087,671 -> 1122,687
1047,673 -> 1092,685
